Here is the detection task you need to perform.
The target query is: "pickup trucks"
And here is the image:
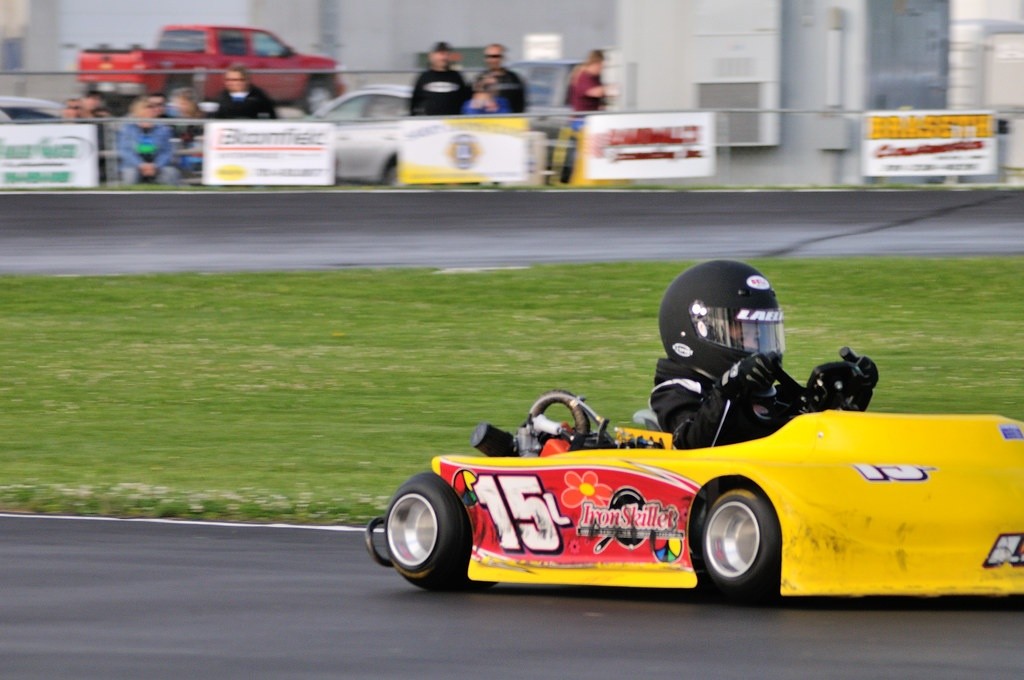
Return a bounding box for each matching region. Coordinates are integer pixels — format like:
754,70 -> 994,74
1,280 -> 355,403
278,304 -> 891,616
79,25 -> 347,116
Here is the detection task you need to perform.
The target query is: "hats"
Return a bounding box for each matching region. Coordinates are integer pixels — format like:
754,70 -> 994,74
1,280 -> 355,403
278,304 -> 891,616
432,42 -> 452,52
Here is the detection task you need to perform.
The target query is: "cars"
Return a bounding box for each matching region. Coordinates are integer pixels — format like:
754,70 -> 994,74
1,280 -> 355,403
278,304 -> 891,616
310,84 -> 415,186
503,61 -> 585,167
1,97 -> 67,122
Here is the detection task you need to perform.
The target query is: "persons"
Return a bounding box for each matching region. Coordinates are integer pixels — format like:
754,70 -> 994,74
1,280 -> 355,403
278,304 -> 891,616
207,64 -> 278,122
409,42 -> 472,116
461,77 -> 512,113
477,43 -> 526,111
58,86 -> 208,186
651,260 -> 878,449
560,50 -> 620,181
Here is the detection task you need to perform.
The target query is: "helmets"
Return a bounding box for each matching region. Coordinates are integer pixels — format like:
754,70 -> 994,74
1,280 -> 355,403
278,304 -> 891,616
657,259 -> 787,408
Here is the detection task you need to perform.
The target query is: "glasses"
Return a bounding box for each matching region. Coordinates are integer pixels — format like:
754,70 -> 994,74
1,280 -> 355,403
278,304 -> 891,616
223,77 -> 246,82
65,104 -> 80,110
484,53 -> 502,59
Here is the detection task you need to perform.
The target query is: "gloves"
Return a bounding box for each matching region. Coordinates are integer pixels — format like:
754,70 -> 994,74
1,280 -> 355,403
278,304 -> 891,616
716,350 -> 783,406
849,355 -> 879,391
